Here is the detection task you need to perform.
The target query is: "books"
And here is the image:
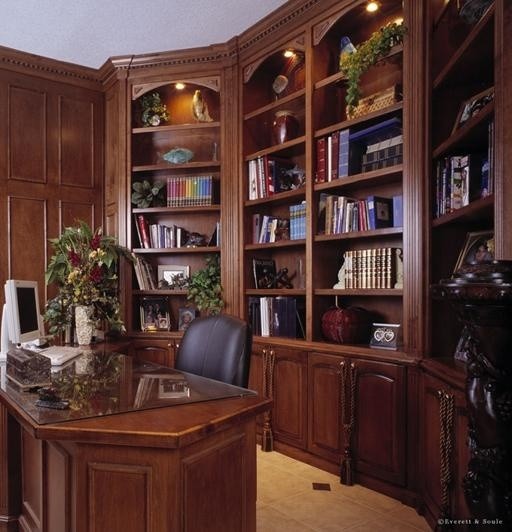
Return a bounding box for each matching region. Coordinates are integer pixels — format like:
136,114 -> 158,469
317,120 -> 403,184
249,296 -> 296,340
166,176 -> 214,207
317,193 -> 403,235
249,156 -> 293,200
253,259 -> 277,288
132,377 -> 154,409
135,215 -> 182,249
207,223 -> 219,247
133,255 -> 157,290
437,155 -> 489,218
345,248 -> 398,289
253,203 -> 306,244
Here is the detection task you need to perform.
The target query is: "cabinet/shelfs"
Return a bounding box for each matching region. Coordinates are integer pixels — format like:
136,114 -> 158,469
234,3 -> 423,504
417,4 -> 510,531
106,45 -> 240,372
105,4 -> 511,531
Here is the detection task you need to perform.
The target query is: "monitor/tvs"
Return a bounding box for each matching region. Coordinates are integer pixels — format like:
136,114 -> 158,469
0,279 -> 44,362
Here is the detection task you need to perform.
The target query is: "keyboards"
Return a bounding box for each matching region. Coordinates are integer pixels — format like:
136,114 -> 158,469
39,346 -> 84,366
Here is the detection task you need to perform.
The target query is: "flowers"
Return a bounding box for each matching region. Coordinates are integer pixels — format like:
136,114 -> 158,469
48,221 -> 137,336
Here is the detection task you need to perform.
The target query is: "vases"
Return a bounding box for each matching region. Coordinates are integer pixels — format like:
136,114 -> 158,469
75,306 -> 93,345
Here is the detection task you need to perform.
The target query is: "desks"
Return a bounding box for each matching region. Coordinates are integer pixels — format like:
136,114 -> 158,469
1,342 -> 274,531
